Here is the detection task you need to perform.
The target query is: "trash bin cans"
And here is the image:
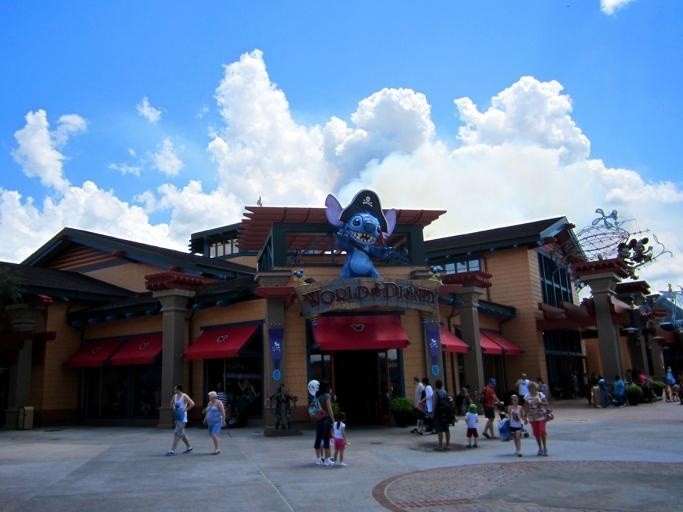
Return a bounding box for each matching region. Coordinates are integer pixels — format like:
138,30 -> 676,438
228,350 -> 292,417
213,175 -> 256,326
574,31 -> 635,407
24,406 -> 35,430
591,386 -> 600,408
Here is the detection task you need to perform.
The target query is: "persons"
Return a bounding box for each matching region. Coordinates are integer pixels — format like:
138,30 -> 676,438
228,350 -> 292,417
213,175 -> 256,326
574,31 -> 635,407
407,369 -> 553,456
163,384 -> 195,456
330,410 -> 348,466
201,390 -> 225,455
571,364 -> 683,408
308,380 -> 335,466
268,384 -> 296,430
216,375 -> 263,428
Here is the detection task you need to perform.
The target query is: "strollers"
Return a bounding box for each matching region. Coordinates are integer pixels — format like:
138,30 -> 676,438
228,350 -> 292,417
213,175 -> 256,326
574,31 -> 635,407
494,399 -> 529,441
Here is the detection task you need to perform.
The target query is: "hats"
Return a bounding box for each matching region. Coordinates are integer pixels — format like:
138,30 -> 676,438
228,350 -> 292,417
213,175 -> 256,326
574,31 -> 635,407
489,377 -> 497,385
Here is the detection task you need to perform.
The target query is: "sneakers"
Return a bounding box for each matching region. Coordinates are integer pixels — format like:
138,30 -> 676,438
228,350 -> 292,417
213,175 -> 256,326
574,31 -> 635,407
315,456 -> 348,467
167,447 -> 221,456
537,447 -> 549,456
482,431 -> 490,439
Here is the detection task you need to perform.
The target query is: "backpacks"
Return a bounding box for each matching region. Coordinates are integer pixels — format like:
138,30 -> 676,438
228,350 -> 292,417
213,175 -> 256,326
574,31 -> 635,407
307,395 -> 327,422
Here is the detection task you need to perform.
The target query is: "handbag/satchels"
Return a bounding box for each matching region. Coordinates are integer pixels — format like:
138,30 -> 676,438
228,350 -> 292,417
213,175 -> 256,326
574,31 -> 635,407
543,406 -> 555,422
478,385 -> 487,403
